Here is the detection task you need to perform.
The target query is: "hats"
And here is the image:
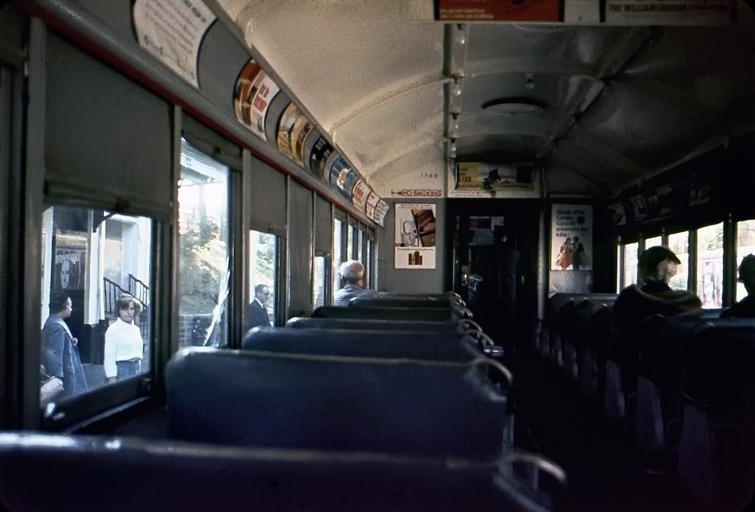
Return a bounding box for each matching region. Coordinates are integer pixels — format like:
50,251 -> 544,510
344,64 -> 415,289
339,260 -> 364,279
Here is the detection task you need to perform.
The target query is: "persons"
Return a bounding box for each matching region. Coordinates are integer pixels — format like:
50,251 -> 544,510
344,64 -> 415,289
719,255 -> 754,319
41,292 -> 89,404
612,247 -> 680,325
248,284 -> 271,331
102,293 -> 143,385
571,236 -> 585,270
560,238 -> 572,270
335,259 -> 379,307
59,260 -> 77,290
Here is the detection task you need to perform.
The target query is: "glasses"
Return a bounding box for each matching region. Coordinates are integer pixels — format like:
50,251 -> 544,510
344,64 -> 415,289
261,292 -> 269,296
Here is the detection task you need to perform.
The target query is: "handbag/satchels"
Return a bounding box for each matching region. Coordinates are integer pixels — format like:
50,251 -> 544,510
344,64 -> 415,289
40,372 -> 65,410
